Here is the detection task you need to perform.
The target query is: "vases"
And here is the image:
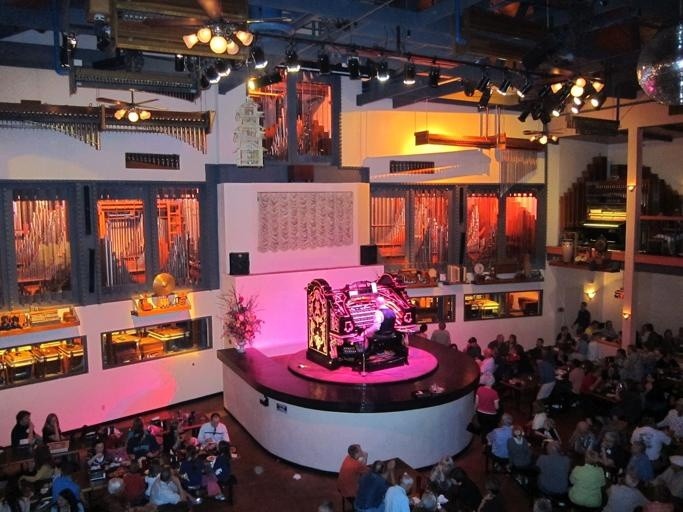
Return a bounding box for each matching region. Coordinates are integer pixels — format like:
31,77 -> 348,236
236,341 -> 247,353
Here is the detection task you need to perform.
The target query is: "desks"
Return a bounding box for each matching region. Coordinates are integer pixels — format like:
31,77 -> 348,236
368,457 -> 427,492
29,469 -> 109,504
500,373 -> 538,411
586,390 -> 618,405
0,410 -> 209,465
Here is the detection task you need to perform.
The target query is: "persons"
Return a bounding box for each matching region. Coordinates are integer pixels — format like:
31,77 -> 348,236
336,444 -> 370,500
656,396 -> 683,438
465,335 -> 482,356
449,342 -> 459,353
505,425 -> 530,483
432,322 -> 451,343
17,447 -> 56,481
200,460 -> 221,503
145,459 -> 161,496
179,445 -> 200,494
101,479 -> 130,512
354,462 -> 389,511
529,338 -> 549,359
580,364 -> 602,393
149,468 -> 185,505
568,358 -> 588,393
49,488 -> 85,512
567,421 -> 594,458
210,441 -> 232,488
536,441 -> 571,495
532,499 -> 552,512
534,418 -> 563,448
197,413 -> 231,446
486,334 -> 504,350
637,487 -> 681,512
439,469 -> 480,512
595,434 -> 620,464
477,492 -> 494,512
0,481 -> 32,512
649,455 -> 683,499
603,470 -> 644,511
501,335 -> 523,363
630,416 -> 672,459
430,457 -> 453,488
570,453 -> 609,507
414,323 -> 428,340
472,372 -> 499,436
609,323 -> 683,397
585,315 -> 599,335
590,368 -> 610,397
557,325 -> 577,350
11,410 -> 41,448
535,347 -> 558,399
85,440 -> 115,485
315,501 -> 334,512
32,478 -> 52,511
358,297 -> 396,359
162,420 -> 188,454
115,461 -> 146,505
384,474 -> 410,512
528,400 -> 548,432
475,348 -> 498,374
484,415 -> 517,477
625,441 -> 653,482
42,413 -> 63,443
125,418 -> 160,451
570,300 -> 588,332
602,319 -> 617,340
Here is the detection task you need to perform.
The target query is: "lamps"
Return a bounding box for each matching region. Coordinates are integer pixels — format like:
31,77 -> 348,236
285,51 -> 300,72
591,91 -> 607,111
182,22 -> 254,57
497,78 -> 512,96
478,88 -> 493,110
538,83 -> 550,98
428,66 -> 439,88
403,64 -> 416,85
529,134 -> 559,145
377,60 -> 389,82
348,58 -> 361,80
477,76 -> 492,92
114,107 -> 151,123
174,54 -> 185,73
251,47 -> 264,69
319,55 -> 330,76
552,104 -> 565,119
215,61 -> 230,77
517,109 -> 529,123
516,79 -> 532,98
204,68 -> 220,84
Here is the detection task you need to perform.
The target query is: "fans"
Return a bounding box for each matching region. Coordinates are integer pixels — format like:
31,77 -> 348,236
140,0 -> 297,37
523,124 -> 564,136
95,90 -> 160,110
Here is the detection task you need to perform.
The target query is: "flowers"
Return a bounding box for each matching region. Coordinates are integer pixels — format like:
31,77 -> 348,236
217,284 -> 264,345
